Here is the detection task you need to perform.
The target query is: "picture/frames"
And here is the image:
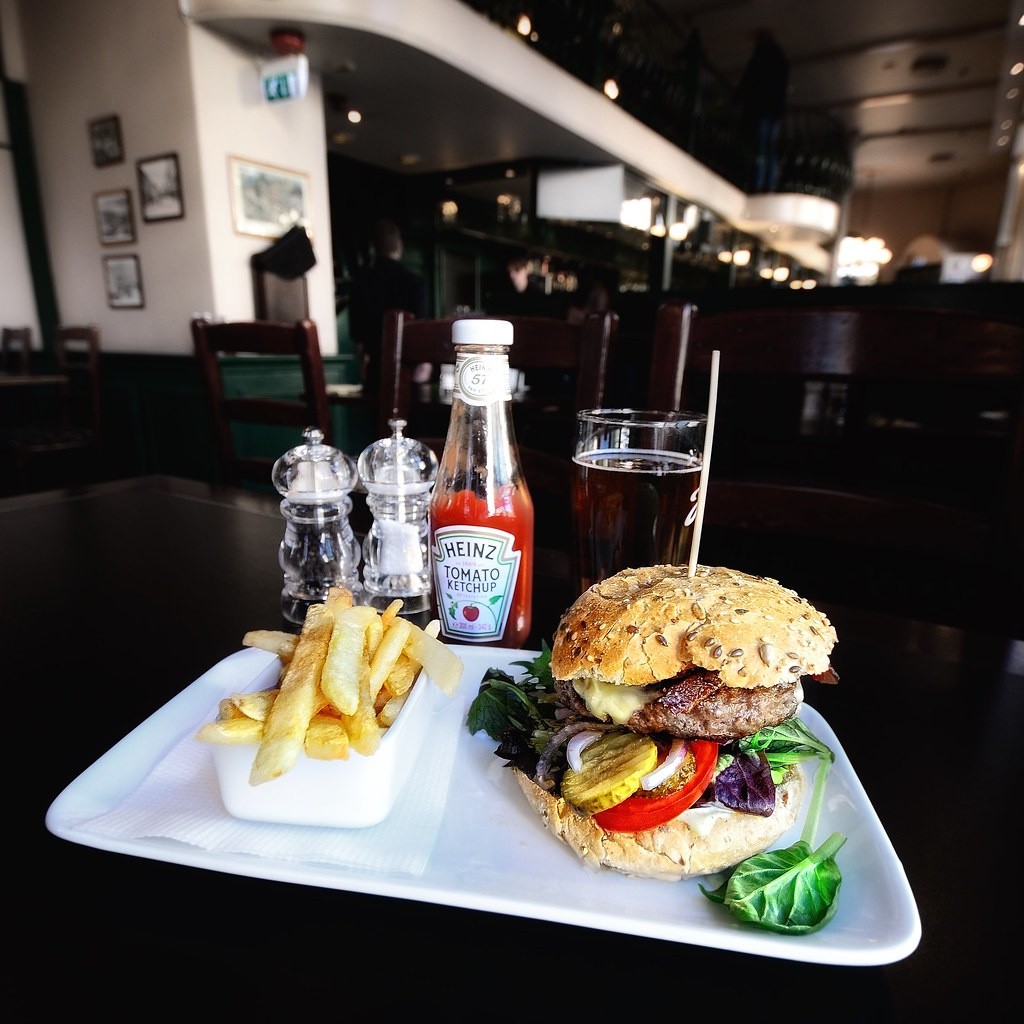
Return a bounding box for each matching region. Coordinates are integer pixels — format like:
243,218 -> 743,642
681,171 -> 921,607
92,189 -> 137,246
101,252 -> 144,310
136,153 -> 186,223
88,115 -> 124,169
227,152 -> 315,248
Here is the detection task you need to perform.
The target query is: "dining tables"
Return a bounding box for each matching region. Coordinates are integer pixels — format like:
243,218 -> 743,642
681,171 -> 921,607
0,371 -> 70,477
0,473 -> 1024,1024
301,384 -> 564,455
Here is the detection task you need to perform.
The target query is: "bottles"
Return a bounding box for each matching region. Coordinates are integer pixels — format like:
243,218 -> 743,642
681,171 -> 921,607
427,318 -> 535,648
352,417 -> 440,615
271,424 -> 362,629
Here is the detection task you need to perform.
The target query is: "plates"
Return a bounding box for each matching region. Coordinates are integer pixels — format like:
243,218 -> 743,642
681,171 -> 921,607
41,643 -> 922,971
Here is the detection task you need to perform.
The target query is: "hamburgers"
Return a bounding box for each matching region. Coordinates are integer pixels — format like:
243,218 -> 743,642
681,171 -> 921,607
465,563 -> 837,880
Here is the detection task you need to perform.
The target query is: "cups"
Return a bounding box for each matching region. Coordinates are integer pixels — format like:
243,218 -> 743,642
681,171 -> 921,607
572,406 -> 710,596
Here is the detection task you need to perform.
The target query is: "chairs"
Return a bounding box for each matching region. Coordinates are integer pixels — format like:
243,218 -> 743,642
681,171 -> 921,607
380,307 -> 617,551
190,316 -> 334,492
641,301 -> 1022,642
20,326 -> 99,490
1,328 -> 31,373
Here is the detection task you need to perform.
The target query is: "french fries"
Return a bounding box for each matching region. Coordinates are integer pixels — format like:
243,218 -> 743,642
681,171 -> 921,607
199,587 -> 463,784
